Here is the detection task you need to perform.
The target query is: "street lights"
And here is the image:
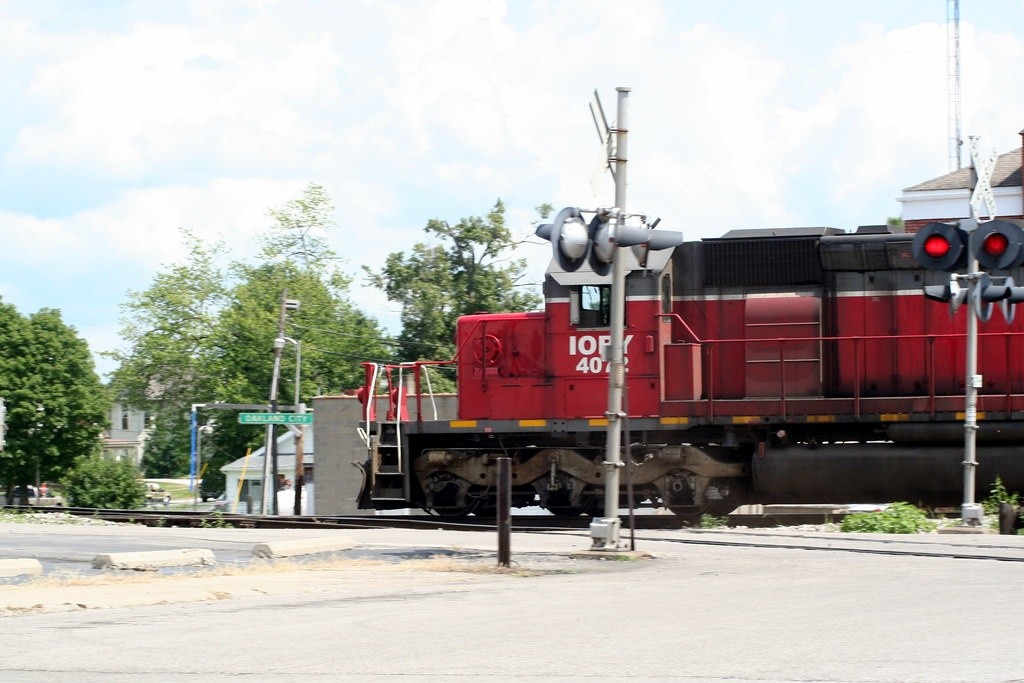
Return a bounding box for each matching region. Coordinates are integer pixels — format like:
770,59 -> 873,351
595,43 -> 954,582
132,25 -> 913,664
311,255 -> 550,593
270,289 -> 299,515
194,426 -> 214,512
273,333 -> 306,515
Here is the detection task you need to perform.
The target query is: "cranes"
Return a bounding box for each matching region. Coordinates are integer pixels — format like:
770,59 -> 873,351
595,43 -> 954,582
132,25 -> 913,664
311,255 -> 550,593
946,0 -> 964,172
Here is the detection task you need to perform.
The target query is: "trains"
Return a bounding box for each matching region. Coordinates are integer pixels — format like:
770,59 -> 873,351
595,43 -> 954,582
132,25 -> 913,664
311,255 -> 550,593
351,226 -> 1024,522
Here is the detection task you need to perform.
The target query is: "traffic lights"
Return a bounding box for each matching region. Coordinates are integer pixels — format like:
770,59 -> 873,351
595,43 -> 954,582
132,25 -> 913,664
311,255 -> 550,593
911,222 -> 963,270
631,222 -> 682,269
586,214 -> 613,277
1002,276 -> 1024,324
971,220 -> 1021,269
616,224 -> 652,248
536,207 -> 589,272
923,281 -> 962,317
973,272 -> 1012,322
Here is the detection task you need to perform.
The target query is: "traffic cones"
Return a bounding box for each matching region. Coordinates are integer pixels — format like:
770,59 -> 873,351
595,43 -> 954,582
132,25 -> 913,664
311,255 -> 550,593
40,483 -> 47,497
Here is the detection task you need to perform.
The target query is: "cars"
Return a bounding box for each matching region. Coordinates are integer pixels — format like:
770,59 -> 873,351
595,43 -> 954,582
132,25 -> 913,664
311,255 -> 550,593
200,474 -> 225,501
0,485 -> 63,507
145,483 -> 170,503
